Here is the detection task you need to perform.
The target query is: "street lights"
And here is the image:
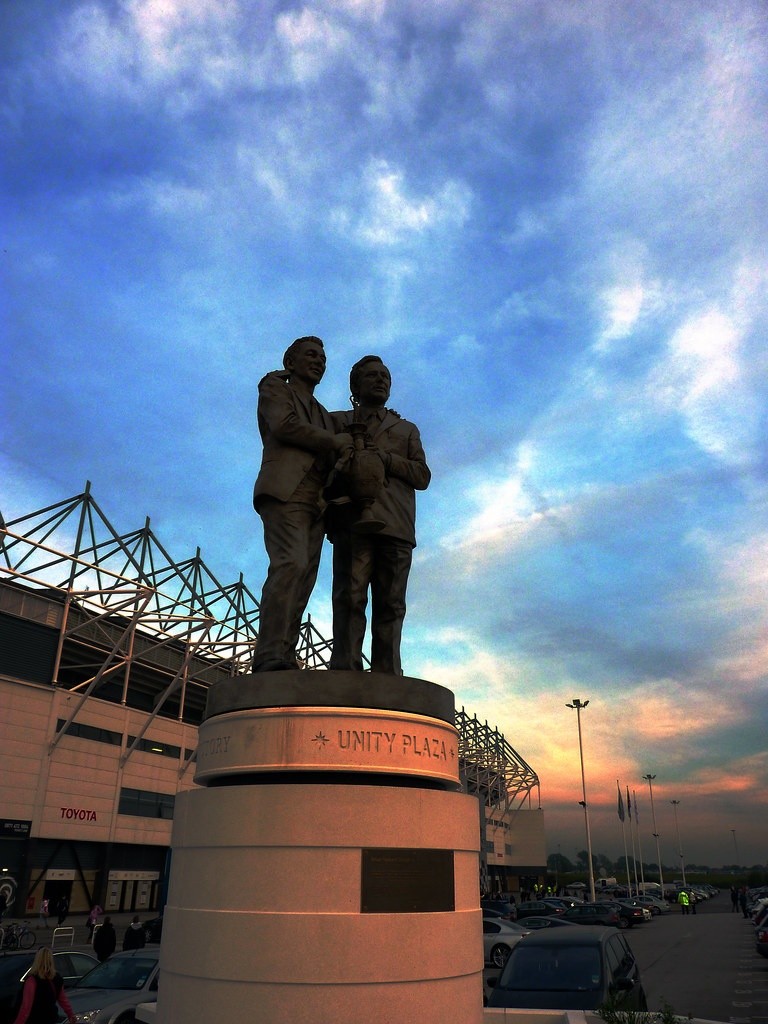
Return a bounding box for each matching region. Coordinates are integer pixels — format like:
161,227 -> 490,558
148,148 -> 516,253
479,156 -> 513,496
730,830 -> 741,868
563,698 -> 598,904
668,799 -> 688,888
642,773 -> 666,898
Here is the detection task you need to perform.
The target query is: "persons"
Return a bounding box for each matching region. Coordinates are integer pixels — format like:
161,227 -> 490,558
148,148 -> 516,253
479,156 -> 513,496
0,891 -> 7,924
11,946 -> 78,1024
731,885 -> 752,919
36,896 -> 49,930
123,916 -> 146,951
57,895 -> 69,927
87,905 -> 102,944
481,884 -> 589,903
94,916 -> 117,961
326,354 -> 431,676
614,888 -> 697,914
253,336 -> 353,672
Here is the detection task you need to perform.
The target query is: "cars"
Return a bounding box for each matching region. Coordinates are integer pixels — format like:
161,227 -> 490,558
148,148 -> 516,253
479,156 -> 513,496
487,925 -> 650,1013
746,886 -> 768,959
56,946 -> 160,1024
0,950 -> 102,993
481,877 -> 720,971
142,914 -> 163,944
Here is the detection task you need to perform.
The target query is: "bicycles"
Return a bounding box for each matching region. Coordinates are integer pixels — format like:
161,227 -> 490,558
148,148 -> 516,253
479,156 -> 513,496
0,920 -> 37,952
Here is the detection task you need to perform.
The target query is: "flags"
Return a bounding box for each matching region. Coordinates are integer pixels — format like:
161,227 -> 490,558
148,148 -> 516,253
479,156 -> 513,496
634,792 -> 639,824
617,783 -> 625,821
627,788 -> 632,821
